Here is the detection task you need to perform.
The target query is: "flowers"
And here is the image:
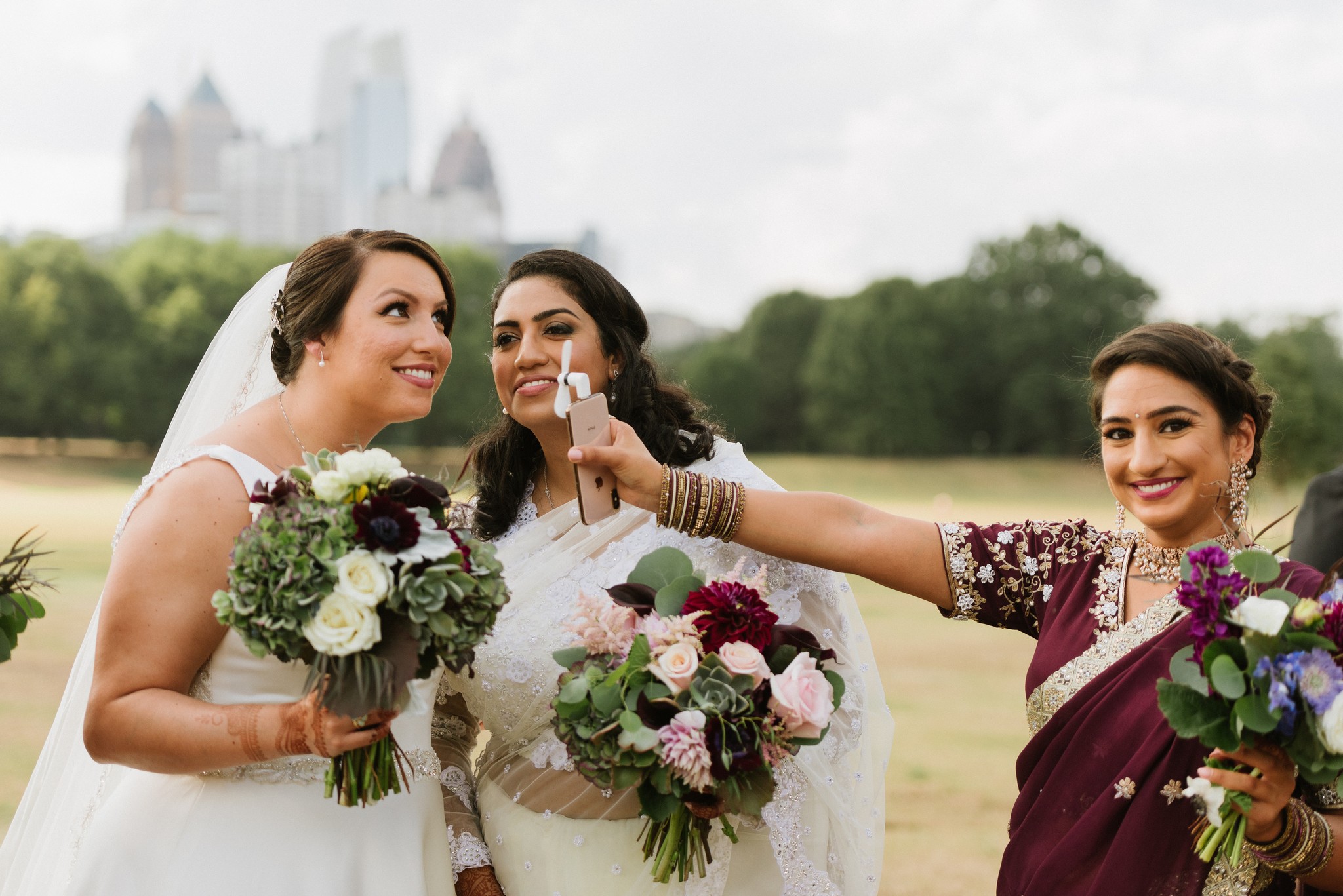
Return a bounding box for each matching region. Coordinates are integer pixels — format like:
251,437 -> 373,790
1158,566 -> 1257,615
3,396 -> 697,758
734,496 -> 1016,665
541,548 -> 854,882
207,447 -> 514,816
1156,537 -> 1343,875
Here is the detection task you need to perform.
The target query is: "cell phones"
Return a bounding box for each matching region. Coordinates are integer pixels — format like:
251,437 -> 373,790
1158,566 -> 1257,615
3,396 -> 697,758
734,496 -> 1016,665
565,391 -> 621,527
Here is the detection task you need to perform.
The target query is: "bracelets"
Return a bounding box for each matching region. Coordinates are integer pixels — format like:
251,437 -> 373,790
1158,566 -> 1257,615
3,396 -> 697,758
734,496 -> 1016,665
655,462 -> 747,545
1241,793 -> 1336,879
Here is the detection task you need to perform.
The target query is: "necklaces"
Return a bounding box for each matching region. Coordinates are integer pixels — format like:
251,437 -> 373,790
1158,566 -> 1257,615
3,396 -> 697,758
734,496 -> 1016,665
277,391 -> 313,458
1132,531 -> 1240,582
543,462 -> 567,535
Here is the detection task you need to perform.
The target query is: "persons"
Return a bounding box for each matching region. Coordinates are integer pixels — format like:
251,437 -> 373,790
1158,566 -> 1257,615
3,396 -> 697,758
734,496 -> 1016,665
560,322 -> 1343,896
427,244 -> 891,894
0,229 -> 464,896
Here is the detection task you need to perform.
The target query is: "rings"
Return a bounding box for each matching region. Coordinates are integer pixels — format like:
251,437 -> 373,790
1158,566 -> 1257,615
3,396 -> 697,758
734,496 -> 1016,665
351,715 -> 367,728
